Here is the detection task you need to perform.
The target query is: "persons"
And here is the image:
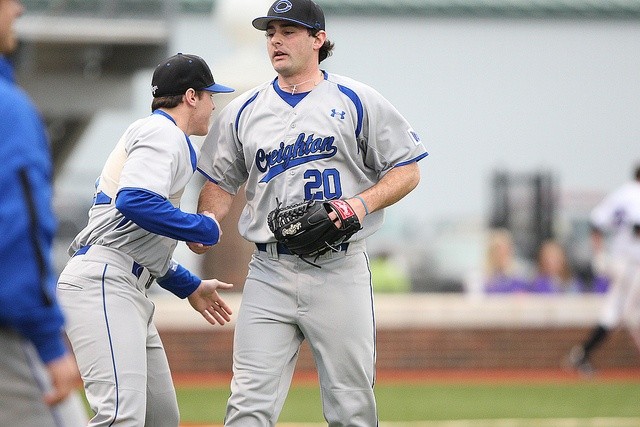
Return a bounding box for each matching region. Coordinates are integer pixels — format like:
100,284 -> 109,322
53,50 -> 235,426
183,1 -> 429,426
1,1 -> 84,426
529,237 -> 583,295
570,163 -> 638,376
467,227 -> 536,294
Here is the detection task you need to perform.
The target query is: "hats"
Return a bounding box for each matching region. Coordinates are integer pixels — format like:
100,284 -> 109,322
151,52 -> 234,98
251,2 -> 326,32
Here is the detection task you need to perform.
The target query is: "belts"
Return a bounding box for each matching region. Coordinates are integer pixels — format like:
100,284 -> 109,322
255,240 -> 348,256
130,261 -> 154,290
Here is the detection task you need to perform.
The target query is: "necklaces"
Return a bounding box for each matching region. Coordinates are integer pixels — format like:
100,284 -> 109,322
277,70 -> 322,95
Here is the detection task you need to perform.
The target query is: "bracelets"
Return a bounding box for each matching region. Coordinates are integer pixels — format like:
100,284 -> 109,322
353,196 -> 369,216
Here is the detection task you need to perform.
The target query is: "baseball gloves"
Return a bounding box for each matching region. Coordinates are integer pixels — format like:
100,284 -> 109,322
267,193 -> 363,269
